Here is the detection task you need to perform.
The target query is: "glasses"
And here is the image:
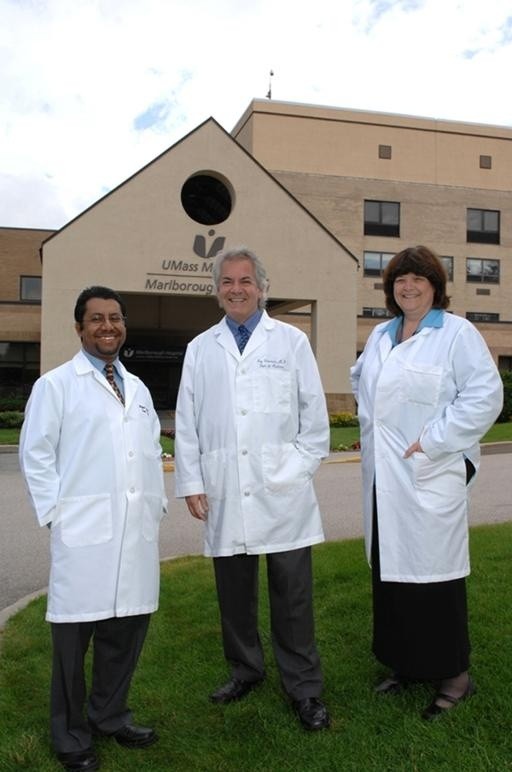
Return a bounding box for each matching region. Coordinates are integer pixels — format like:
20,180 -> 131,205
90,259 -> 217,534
88,313 -> 126,323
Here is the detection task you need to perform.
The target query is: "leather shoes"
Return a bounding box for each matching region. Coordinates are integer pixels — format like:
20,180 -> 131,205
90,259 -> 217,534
207,678 -> 257,705
291,697 -> 331,732
56,749 -> 100,771
108,724 -> 161,748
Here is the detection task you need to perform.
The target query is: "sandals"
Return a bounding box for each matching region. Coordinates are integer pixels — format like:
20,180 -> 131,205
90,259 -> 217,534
372,679 -> 401,695
421,678 -> 478,721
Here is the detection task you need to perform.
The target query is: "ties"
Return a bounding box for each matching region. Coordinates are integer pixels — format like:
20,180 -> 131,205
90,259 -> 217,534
104,363 -> 124,405
238,325 -> 248,355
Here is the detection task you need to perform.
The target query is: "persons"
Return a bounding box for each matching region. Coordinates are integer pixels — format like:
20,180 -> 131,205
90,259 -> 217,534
347,244 -> 506,720
17,286 -> 169,771
172,246 -> 333,733
